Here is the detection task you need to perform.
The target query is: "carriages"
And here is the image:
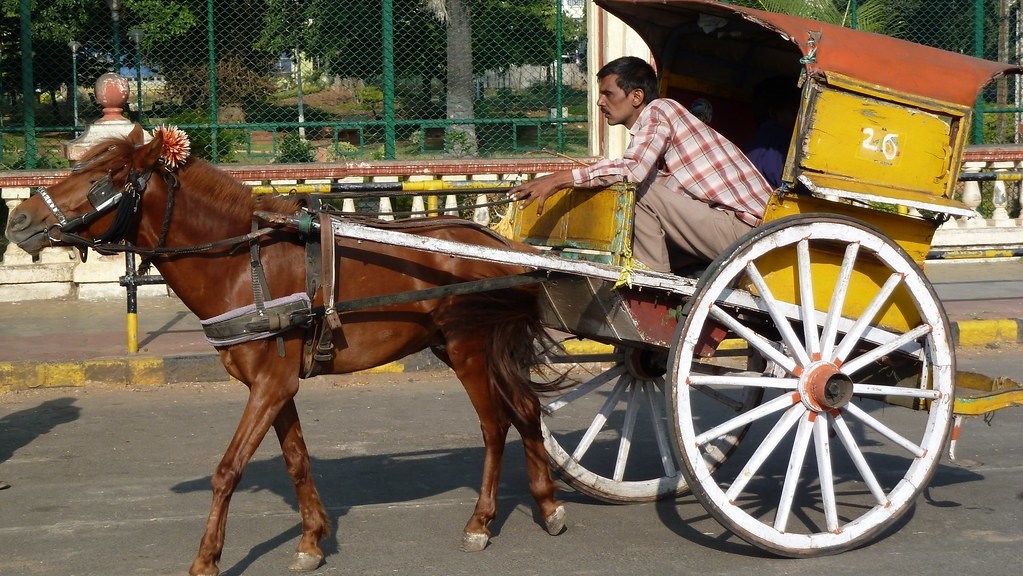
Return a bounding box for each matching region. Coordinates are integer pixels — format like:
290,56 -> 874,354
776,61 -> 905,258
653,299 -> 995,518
1,0 -> 1023,576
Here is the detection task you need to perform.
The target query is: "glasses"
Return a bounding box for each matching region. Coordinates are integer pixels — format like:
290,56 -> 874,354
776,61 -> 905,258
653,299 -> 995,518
774,102 -> 799,114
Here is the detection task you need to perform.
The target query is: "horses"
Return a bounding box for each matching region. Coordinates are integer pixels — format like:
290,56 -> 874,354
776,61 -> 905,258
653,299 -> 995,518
5,121 -> 564,576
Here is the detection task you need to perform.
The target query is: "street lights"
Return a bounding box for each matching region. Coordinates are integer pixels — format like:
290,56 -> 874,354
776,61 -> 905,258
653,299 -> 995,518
125,25 -> 145,128
68,40 -> 81,140
104,0 -> 123,76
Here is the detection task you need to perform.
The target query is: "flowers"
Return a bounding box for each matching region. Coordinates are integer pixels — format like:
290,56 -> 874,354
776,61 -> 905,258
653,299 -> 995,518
152,124 -> 191,168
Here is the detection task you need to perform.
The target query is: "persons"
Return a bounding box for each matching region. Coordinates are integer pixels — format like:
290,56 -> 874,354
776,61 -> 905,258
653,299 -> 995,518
746,75 -> 803,188
506,57 -> 773,276
